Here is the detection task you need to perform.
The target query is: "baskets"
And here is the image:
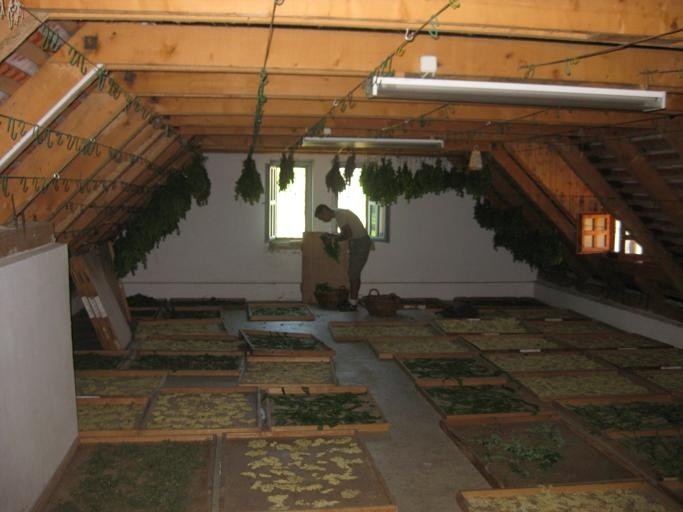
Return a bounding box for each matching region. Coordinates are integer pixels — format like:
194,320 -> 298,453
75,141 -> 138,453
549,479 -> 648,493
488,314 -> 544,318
314,288 -> 350,310
363,295 -> 398,318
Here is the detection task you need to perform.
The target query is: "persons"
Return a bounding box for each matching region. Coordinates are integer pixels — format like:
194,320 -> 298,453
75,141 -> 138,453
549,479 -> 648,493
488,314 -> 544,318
315,204 -> 371,312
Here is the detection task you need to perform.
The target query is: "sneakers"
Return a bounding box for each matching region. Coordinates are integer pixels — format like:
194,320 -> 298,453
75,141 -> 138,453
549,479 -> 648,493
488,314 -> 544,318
338,301 -> 357,312
